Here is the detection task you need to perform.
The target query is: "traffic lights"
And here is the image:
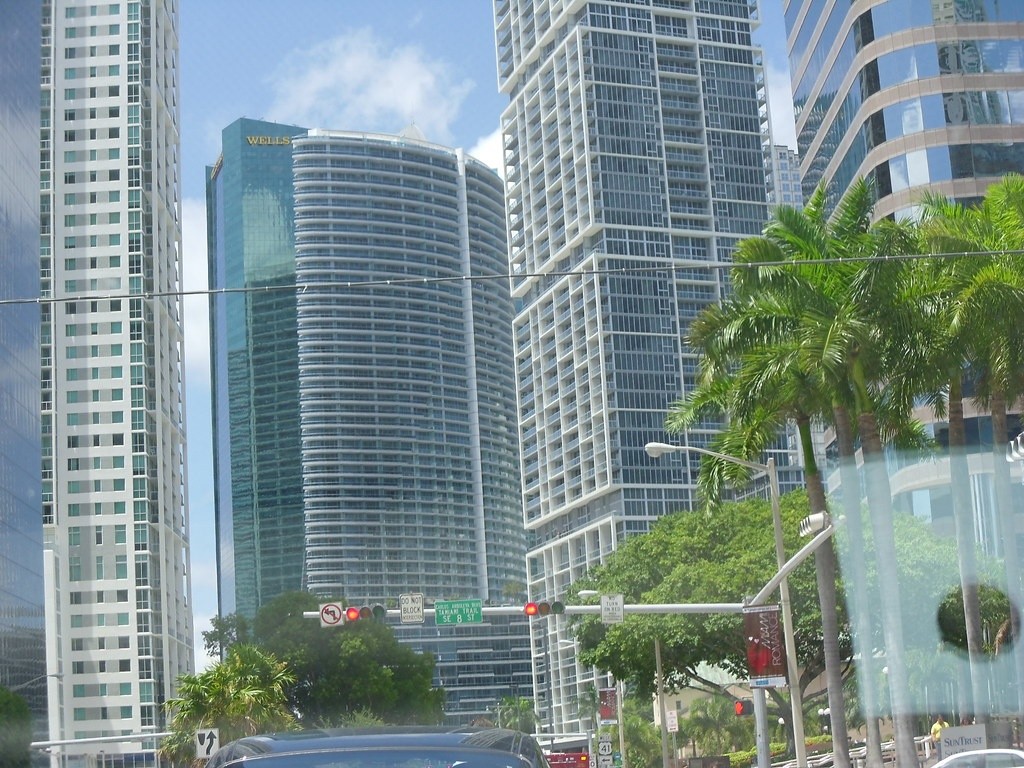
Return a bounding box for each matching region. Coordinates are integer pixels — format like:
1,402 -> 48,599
524,601 -> 564,616
346,603 -> 386,621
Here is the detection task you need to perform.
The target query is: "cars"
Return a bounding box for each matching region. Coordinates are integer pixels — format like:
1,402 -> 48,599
205,726 -> 551,768
930,748 -> 1024,768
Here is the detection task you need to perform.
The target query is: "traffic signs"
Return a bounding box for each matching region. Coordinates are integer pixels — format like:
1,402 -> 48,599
435,599 -> 483,625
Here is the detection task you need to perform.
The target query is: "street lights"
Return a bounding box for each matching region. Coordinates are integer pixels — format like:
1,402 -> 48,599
579,590 -> 669,768
559,639 -> 601,731
645,442 -> 808,768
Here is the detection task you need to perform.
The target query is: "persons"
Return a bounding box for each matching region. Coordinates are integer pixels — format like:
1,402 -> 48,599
960,718 -> 972,726
930,714 -> 950,762
